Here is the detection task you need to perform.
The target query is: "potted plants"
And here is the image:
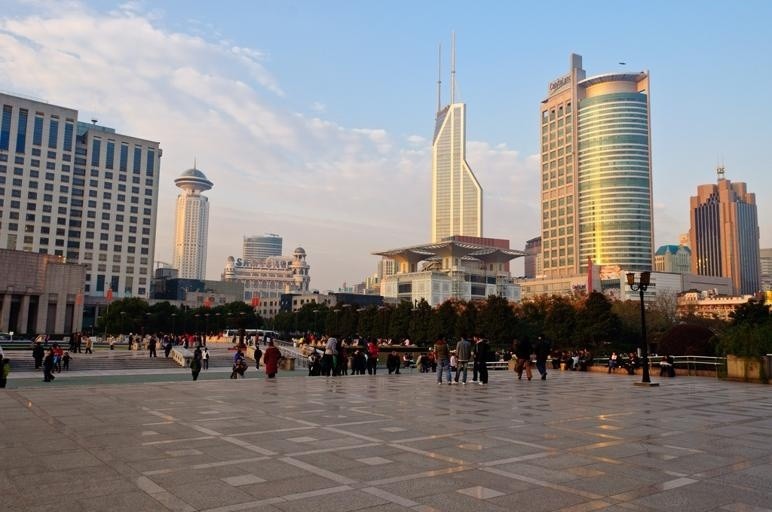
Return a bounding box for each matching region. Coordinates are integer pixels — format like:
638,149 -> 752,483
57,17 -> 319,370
707,318 -> 772,382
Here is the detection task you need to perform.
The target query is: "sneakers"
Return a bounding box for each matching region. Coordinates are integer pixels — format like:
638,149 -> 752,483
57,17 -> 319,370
462,382 -> 466,385
448,381 -> 459,385
479,382 -> 488,386
438,382 -> 442,385
518,375 -> 521,379
469,379 -> 478,384
528,376 -> 532,381
541,372 -> 547,380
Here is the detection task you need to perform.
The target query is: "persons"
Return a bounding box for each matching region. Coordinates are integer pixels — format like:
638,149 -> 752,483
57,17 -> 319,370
493,333 -> 593,380
229,332 -> 491,387
603,348 -> 676,377
31,331 -> 212,383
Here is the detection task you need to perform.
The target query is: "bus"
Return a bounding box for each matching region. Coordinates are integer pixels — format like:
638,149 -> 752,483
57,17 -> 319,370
222,328 -> 266,343
262,331 -> 277,343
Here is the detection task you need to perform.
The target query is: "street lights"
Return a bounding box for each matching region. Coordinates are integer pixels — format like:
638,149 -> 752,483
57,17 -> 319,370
89,325 -> 93,336
626,270 -> 659,387
380,306 -> 387,329
117,310 -> 249,338
313,310 -> 320,333
294,310 -> 299,330
411,308 -> 417,343
334,308 -> 341,334
342,303 -> 353,319
357,308 -> 368,336
